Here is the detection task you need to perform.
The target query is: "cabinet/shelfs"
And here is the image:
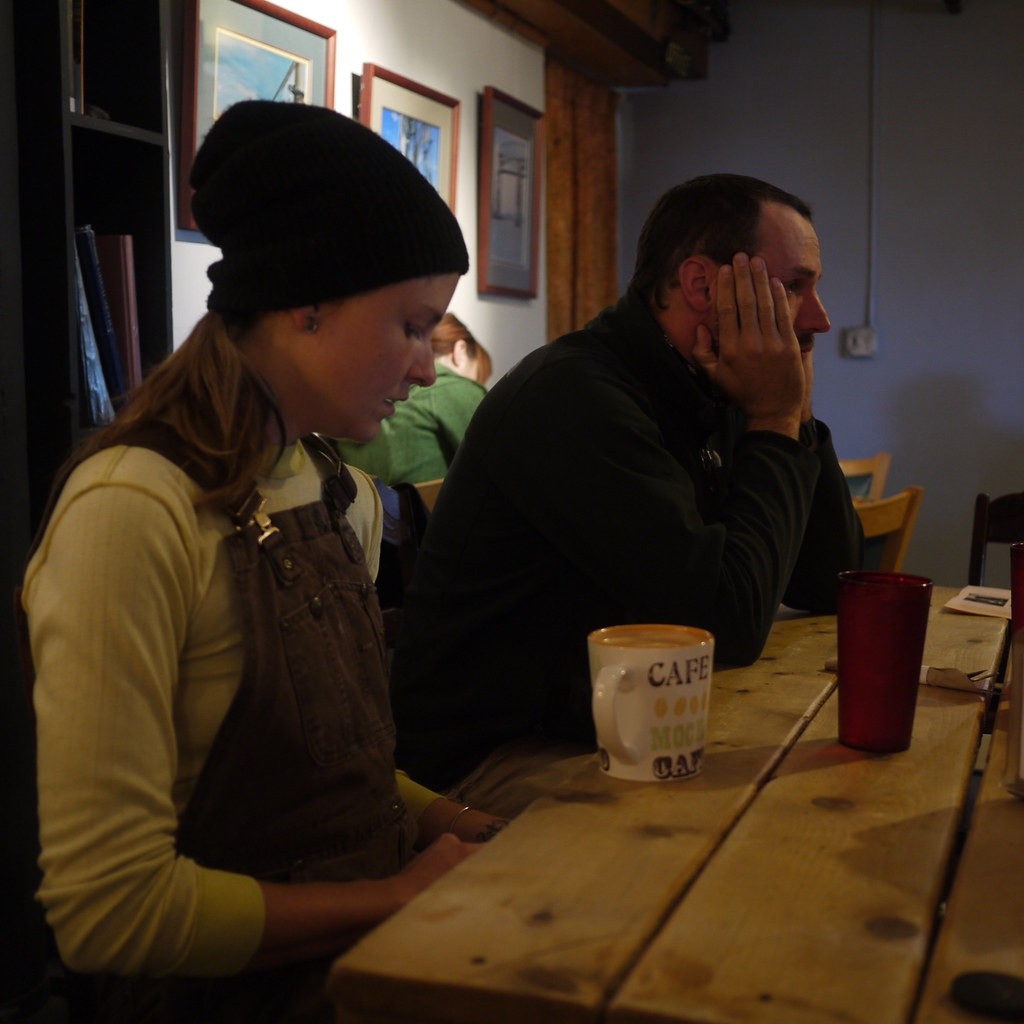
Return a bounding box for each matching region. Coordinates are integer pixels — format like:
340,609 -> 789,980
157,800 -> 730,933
17,0 -> 173,541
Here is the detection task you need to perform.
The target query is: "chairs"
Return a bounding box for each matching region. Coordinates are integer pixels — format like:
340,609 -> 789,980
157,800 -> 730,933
839,452 -> 893,502
853,484 -> 922,574
968,490 -> 1024,588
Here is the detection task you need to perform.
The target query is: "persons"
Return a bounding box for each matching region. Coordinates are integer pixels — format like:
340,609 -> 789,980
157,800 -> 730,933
316,311 -> 489,504
390,172 -> 870,818
0,101 -> 514,1024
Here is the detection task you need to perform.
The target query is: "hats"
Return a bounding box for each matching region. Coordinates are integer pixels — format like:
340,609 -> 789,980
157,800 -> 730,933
190,99 -> 470,317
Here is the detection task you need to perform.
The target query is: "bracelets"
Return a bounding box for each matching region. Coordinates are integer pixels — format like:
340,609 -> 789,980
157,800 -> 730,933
451,805 -> 473,832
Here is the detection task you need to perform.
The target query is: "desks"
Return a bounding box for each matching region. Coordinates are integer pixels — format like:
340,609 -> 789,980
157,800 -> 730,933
331,585 -> 1024,1024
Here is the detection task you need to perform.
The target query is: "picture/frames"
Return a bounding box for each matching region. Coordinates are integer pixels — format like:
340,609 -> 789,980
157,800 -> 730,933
179,0 -> 337,231
475,85 -> 544,296
359,64 -> 458,217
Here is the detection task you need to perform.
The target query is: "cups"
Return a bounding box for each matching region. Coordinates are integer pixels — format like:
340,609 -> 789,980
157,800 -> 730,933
588,625 -> 714,783
837,570 -> 934,752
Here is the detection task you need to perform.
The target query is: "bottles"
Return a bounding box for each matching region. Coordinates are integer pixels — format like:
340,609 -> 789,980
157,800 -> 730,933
1000,542 -> 1024,798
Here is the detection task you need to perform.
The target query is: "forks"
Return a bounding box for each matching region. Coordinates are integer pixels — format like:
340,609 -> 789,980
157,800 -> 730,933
966,669 -> 995,682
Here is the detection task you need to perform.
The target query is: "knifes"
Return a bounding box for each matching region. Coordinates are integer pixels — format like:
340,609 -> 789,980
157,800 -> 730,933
976,687 -> 1003,696
